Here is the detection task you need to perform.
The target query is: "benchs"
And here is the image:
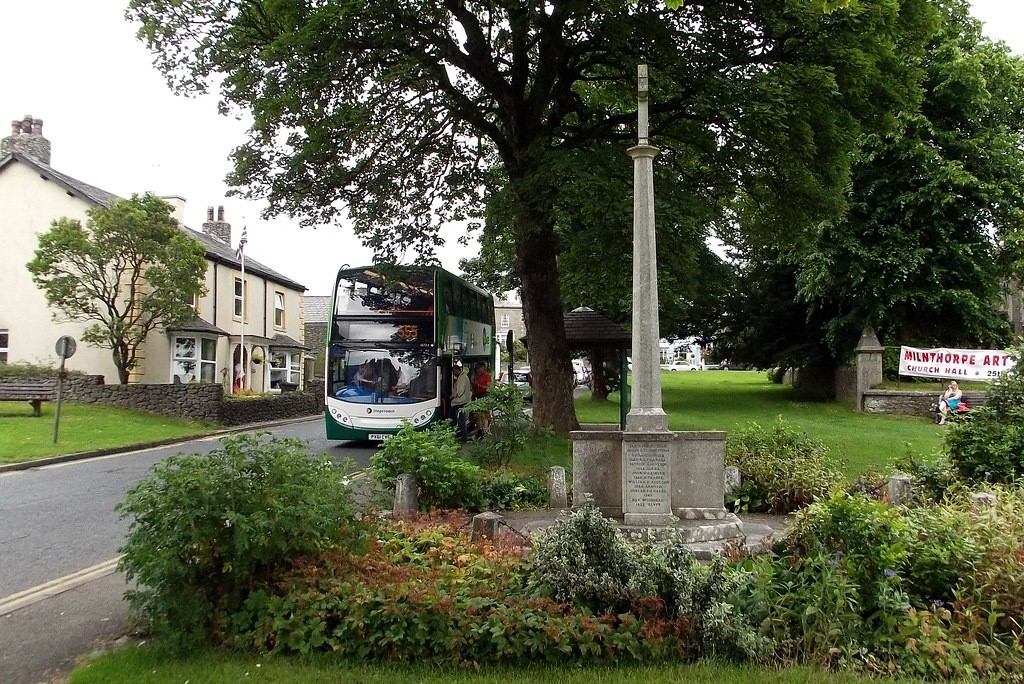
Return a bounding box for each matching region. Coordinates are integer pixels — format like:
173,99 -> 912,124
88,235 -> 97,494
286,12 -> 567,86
933,394 -> 987,424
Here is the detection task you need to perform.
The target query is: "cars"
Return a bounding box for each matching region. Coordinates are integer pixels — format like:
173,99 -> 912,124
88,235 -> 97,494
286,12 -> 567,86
501,371 -> 533,402
571,359 -> 590,385
719,361 -> 753,370
666,360 -> 701,373
522,365 -> 531,372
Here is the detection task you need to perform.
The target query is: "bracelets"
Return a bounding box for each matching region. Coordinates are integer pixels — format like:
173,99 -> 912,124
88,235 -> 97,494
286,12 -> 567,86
450,396 -> 452,399
395,386 -> 397,389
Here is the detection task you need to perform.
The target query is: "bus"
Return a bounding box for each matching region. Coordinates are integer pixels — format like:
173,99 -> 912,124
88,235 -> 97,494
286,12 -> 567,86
322,264 -> 496,441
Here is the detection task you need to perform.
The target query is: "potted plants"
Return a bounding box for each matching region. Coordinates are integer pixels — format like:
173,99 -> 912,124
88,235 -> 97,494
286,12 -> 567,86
251,353 -> 265,365
270,358 -> 282,367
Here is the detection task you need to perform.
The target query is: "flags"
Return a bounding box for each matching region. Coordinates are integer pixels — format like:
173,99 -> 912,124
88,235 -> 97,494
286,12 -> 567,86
234,221 -> 248,261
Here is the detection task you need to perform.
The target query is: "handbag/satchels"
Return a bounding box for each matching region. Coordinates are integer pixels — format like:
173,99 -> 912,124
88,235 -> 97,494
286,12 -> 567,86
233,383 -> 239,392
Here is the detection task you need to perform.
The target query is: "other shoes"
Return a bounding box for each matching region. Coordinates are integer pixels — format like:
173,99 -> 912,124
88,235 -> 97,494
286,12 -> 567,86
458,441 -> 467,444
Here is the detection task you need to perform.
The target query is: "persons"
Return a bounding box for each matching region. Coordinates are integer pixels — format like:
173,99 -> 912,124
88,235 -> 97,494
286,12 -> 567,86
937,381 -> 962,425
234,358 -> 246,393
472,363 -> 492,437
448,365 -> 470,444
352,365 -> 378,390
391,367 -> 428,397
